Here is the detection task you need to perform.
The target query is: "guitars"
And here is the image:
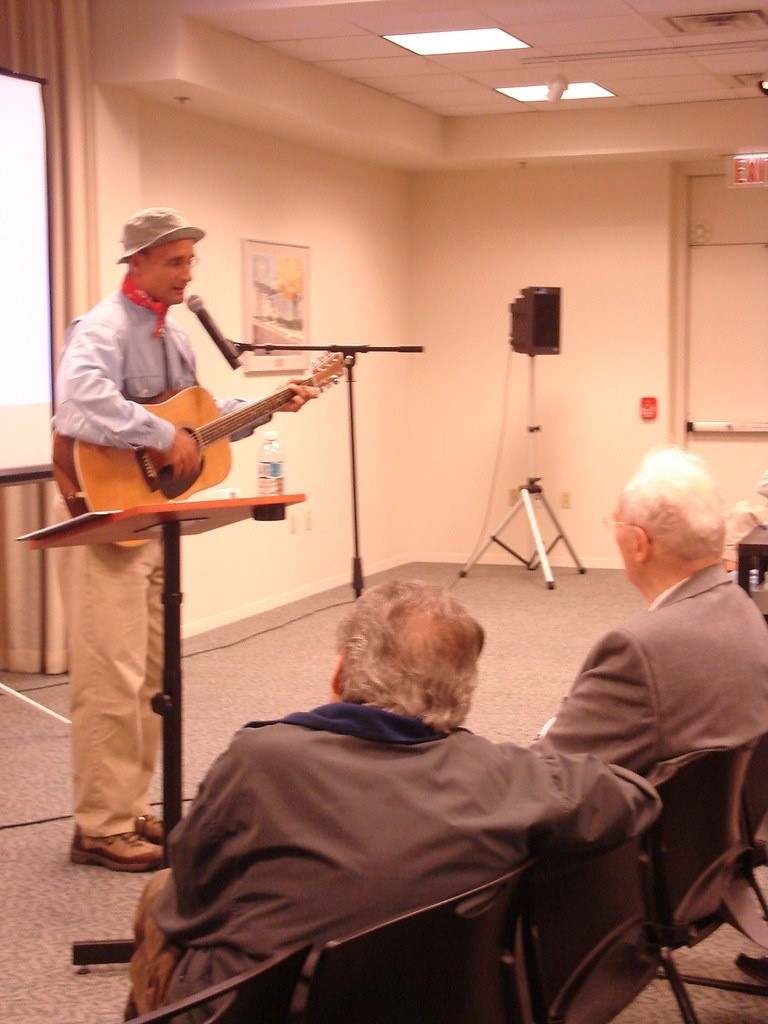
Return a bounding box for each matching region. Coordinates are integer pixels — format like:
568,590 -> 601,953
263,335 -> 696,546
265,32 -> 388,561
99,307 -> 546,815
50,342 -> 353,551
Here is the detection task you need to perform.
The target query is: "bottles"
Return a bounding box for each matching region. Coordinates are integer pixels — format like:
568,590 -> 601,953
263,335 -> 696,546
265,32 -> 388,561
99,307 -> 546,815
258,431 -> 284,496
749,568 -> 759,597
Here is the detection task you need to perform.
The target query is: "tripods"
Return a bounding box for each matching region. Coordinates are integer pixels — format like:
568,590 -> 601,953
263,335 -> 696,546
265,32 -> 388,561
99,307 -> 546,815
460,355 -> 584,590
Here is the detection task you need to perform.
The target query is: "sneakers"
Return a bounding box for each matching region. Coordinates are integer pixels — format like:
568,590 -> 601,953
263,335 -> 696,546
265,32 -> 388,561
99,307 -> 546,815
137,814 -> 165,844
71,833 -> 163,871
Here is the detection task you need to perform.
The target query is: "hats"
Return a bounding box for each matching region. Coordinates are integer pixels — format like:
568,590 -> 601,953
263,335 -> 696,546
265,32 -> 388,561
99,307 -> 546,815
117,207 -> 204,263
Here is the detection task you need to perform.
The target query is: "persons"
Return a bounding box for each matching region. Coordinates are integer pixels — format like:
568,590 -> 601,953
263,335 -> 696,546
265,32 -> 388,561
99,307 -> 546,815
56,207 -> 322,872
524,451 -> 768,849
124,581 -> 658,1024
722,469 -> 768,586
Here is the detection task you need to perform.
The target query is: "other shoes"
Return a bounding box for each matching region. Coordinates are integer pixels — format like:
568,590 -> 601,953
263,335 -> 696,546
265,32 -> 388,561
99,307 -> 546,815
734,952 -> 768,983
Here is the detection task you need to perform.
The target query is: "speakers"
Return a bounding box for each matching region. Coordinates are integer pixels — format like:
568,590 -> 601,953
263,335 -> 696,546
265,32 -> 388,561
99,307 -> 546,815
508,287 -> 561,357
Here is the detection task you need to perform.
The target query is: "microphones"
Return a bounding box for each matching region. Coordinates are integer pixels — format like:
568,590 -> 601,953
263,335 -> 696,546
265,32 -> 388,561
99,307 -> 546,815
187,295 -> 243,370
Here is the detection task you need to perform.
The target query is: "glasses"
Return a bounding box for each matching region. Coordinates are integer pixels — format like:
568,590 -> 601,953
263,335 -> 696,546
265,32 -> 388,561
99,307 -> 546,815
602,510 -> 652,546
145,256 -> 199,271
